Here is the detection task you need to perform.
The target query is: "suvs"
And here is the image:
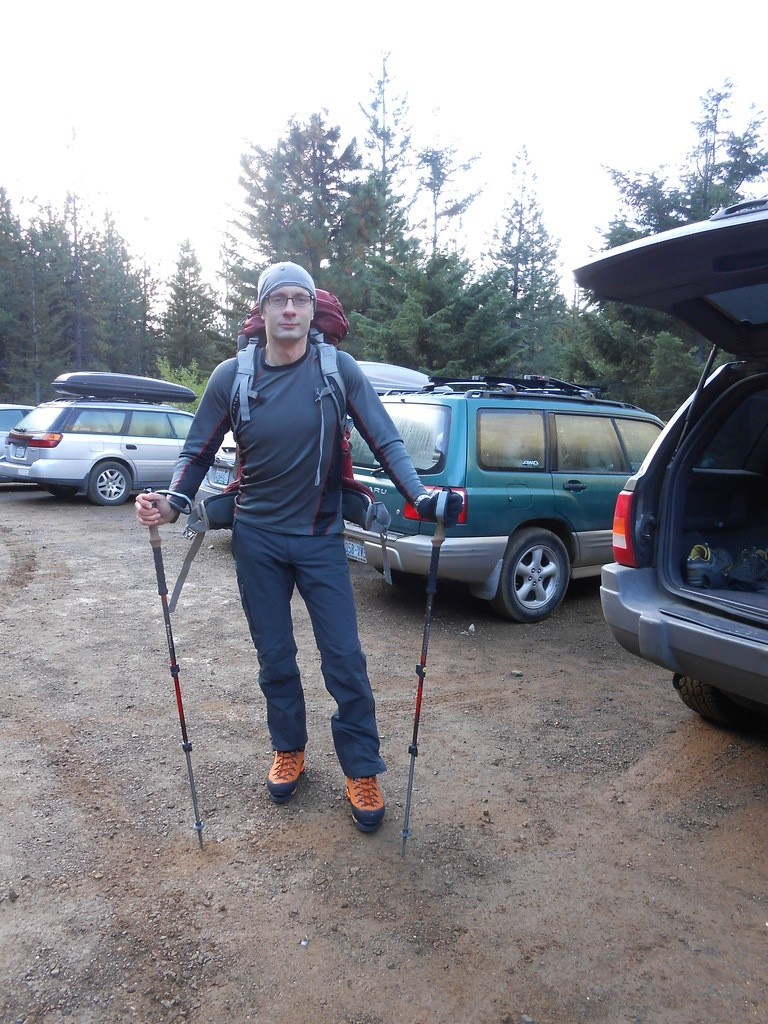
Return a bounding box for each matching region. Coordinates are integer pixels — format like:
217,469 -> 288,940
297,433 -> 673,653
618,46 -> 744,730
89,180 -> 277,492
340,372 -> 715,622
3,371 -> 213,507
194,361 -> 454,527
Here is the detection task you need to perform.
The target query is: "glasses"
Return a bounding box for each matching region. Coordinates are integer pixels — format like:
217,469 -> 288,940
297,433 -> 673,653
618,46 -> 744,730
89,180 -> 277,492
266,294 -> 314,310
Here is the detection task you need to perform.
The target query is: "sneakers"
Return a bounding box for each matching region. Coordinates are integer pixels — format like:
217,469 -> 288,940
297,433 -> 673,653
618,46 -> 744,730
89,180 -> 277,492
267,750 -> 305,803
687,542 -> 732,585
728,546 -> 768,582
346,775 -> 385,832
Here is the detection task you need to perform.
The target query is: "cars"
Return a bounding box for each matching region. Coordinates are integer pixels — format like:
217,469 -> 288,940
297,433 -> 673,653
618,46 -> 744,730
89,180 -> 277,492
571,192 -> 768,722
0,404 -> 46,484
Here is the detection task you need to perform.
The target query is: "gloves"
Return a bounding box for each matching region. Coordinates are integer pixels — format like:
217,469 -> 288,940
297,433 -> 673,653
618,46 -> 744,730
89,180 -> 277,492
417,487 -> 463,528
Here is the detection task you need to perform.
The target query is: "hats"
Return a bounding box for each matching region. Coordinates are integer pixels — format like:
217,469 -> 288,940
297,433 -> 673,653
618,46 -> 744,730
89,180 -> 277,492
257,262 -> 316,313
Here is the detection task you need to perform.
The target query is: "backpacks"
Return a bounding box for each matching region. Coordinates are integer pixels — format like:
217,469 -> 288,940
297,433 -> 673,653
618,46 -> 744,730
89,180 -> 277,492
181,288 -> 391,539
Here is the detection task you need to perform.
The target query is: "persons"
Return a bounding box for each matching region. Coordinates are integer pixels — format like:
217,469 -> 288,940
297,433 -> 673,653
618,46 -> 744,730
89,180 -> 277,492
134,261 -> 467,833
